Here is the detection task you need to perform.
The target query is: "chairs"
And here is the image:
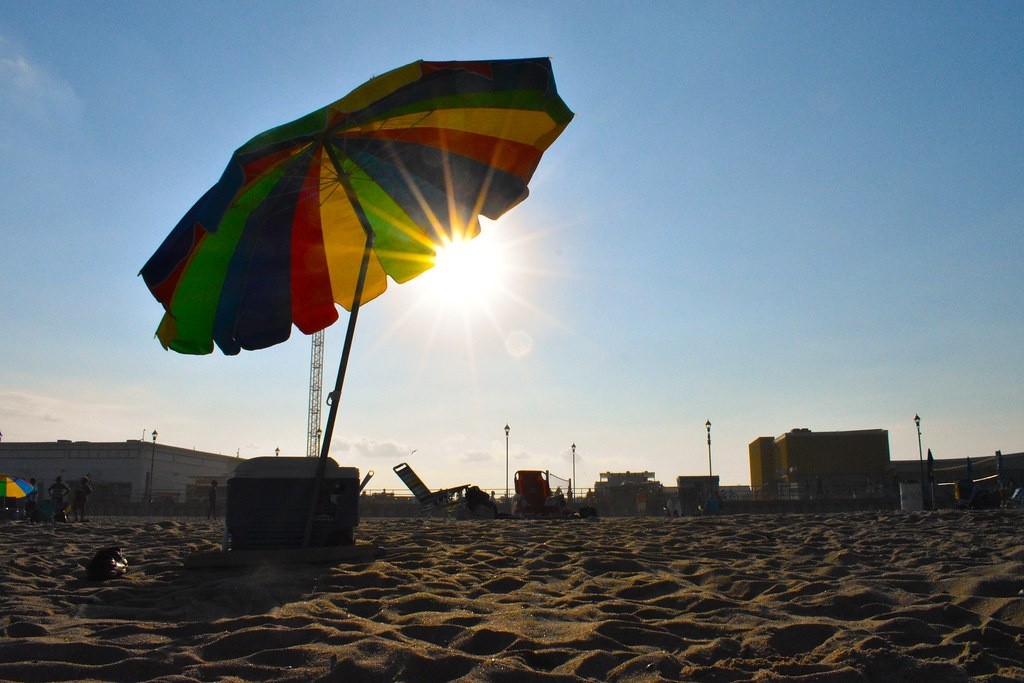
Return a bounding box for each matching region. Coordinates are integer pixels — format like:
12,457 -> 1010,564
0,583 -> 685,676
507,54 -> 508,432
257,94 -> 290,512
512,470 -> 551,517
391,463 -> 472,531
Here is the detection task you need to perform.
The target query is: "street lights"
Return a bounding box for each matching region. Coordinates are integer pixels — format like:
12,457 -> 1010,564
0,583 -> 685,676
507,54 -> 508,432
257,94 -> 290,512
504,424 -> 510,498
571,444 -> 576,502
705,420 -> 711,497
148,430 -> 158,502
915,415 -> 927,504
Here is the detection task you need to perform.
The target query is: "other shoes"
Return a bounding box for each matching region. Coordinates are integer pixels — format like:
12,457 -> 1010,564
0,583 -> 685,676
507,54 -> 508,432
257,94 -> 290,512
86,547 -> 128,580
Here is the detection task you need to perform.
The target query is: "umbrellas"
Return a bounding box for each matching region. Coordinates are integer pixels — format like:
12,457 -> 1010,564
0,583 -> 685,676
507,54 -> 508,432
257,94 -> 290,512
0,473 -> 34,506
137,57 -> 575,546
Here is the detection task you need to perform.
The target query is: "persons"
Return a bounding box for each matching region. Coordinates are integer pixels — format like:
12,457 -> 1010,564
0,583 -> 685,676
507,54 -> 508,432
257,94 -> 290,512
207,480 -> 218,520
72,476 -> 92,523
47,476 -> 69,507
26,478 -> 38,505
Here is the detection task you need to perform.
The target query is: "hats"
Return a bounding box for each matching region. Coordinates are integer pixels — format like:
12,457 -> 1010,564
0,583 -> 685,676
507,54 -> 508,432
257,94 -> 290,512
56,476 -> 62,481
